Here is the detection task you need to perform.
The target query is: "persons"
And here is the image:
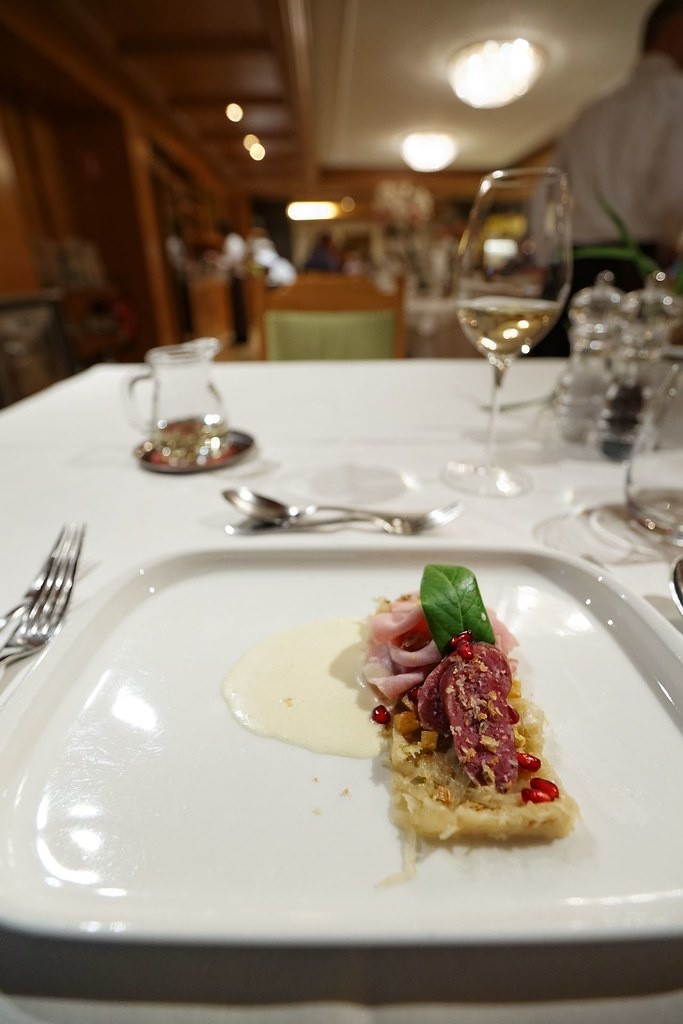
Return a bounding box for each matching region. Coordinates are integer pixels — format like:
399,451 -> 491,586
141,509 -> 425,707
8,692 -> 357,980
522,0 -> 683,373
54,195 -> 533,375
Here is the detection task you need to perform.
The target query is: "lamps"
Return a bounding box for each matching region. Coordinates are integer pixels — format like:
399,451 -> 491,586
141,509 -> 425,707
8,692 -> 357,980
448,36 -> 552,109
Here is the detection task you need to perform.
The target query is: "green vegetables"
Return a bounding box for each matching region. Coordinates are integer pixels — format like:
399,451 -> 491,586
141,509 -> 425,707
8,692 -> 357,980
418,563 -> 496,659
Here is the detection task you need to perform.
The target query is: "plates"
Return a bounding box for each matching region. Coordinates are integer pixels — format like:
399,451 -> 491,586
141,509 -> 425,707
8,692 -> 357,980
2,535 -> 683,943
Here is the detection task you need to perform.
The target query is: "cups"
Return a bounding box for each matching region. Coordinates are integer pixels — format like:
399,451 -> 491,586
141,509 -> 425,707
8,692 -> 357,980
119,334 -> 231,467
625,365 -> 683,535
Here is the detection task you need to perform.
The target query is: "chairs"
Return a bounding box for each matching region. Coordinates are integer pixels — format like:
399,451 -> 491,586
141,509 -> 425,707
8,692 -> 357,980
256,262 -> 407,359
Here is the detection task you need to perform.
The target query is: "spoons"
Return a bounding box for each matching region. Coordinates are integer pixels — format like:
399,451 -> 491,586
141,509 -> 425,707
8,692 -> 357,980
225,485 -> 434,525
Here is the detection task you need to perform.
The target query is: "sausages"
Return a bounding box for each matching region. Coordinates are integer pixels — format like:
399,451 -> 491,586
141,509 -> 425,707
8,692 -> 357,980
418,640 -> 516,787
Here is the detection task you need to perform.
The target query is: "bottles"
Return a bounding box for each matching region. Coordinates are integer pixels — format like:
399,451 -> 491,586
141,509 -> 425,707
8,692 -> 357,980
553,264 -> 683,460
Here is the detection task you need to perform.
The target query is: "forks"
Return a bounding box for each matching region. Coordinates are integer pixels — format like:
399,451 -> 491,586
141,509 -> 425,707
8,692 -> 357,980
226,500 -> 464,536
1,523 -> 87,671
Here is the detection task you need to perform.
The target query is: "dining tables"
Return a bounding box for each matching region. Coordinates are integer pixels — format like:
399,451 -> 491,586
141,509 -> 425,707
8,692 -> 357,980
0,362 -> 682,1024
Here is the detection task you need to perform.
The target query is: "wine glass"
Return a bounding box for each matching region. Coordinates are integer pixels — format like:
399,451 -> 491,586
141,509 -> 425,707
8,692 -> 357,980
435,163 -> 575,498
